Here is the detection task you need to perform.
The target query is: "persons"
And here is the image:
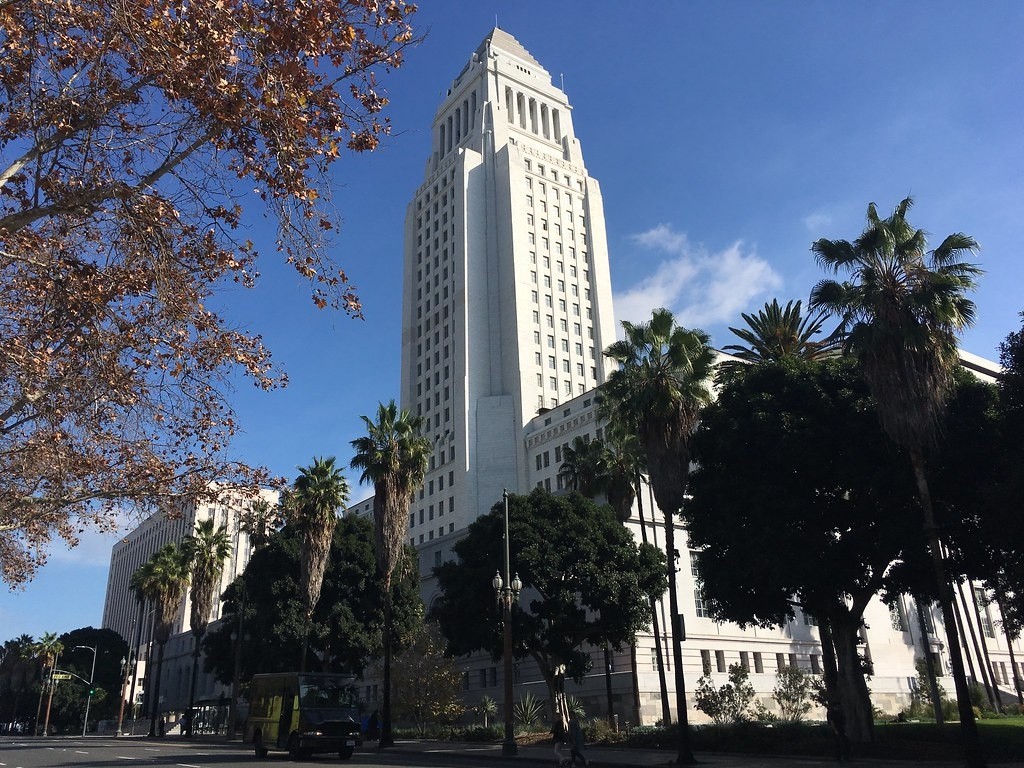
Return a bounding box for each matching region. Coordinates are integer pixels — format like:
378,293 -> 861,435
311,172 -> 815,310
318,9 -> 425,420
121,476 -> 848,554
549,714 -> 566,766
178,716 -> 186,735
157,716 -> 165,737
568,711 -> 589,768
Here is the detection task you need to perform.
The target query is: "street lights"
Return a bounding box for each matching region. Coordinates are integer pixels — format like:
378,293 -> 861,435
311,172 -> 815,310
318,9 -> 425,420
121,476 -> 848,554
76,646 -> 97,737
114,655 -> 136,736
493,569 -> 522,755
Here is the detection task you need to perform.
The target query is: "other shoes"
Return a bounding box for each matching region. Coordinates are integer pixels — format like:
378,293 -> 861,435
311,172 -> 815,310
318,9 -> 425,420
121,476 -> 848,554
585,759 -> 590,767
571,763 -> 576,767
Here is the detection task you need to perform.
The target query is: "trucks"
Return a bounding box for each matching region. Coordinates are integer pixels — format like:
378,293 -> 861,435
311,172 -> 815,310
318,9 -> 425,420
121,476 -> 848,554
243,671 -> 362,761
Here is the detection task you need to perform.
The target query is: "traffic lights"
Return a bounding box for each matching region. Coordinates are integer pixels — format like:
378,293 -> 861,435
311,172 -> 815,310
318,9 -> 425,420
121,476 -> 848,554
43,665 -> 52,681
89,683 -> 95,696
